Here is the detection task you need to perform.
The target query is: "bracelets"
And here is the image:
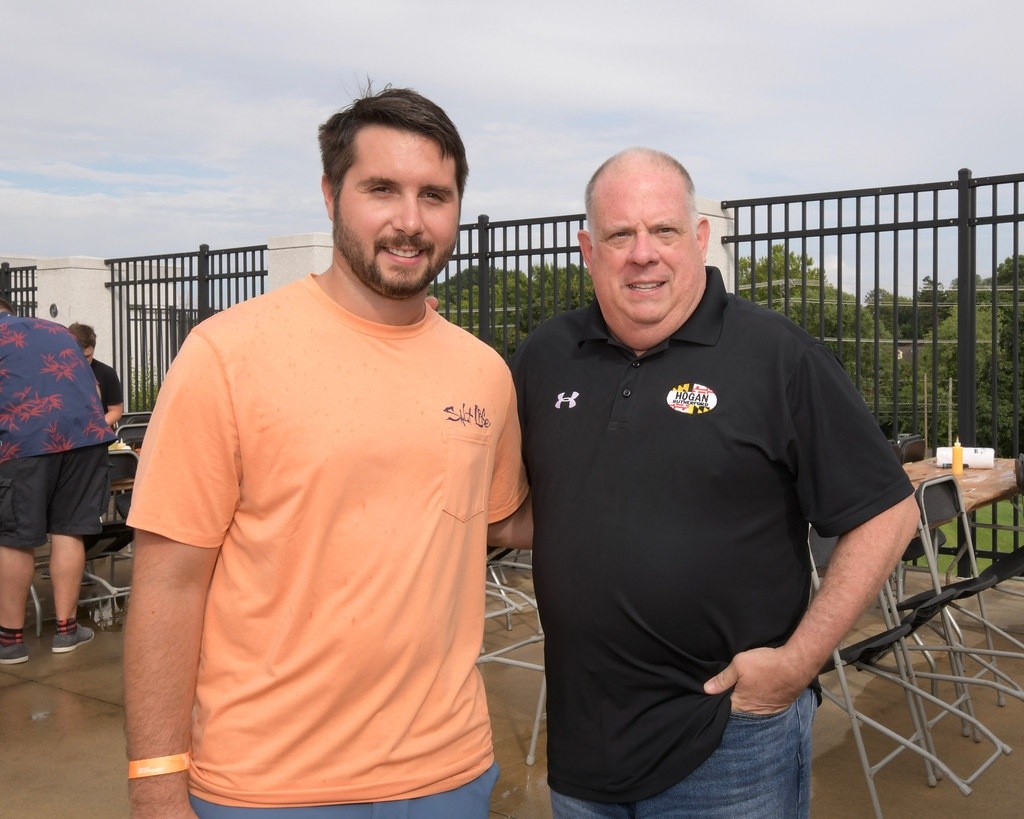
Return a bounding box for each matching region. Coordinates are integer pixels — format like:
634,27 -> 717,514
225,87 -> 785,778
127,753 -> 189,780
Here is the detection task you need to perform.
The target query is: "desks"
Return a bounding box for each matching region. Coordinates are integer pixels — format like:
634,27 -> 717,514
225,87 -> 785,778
903,455 -> 1022,626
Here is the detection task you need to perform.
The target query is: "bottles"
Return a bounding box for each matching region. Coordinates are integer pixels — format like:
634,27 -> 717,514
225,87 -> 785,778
118,438 -> 126,451
952,436 -> 963,476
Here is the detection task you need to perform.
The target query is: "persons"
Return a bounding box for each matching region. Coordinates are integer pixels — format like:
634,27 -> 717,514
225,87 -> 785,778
123,90 -> 535,819
512,150 -> 920,819
41,323 -> 123,584
0,298 -> 118,664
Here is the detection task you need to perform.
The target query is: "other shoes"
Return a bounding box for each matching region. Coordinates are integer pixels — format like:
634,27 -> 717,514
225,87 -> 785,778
0,644 -> 29,664
52,623 -> 94,653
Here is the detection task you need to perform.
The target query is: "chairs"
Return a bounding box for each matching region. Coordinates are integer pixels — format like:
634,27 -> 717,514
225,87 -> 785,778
808,475 -> 1024,819
29,411 -> 153,638
